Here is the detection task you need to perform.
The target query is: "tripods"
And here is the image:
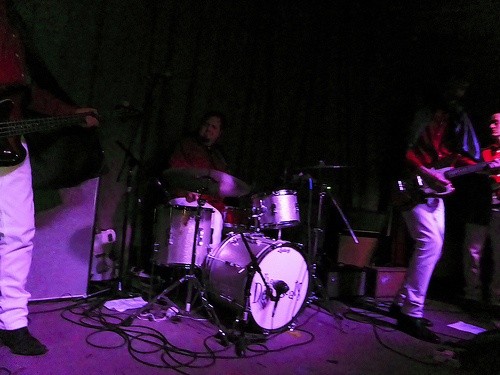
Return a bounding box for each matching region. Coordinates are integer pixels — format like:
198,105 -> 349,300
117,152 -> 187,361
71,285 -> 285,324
310,183 -> 360,320
72,139 -> 229,347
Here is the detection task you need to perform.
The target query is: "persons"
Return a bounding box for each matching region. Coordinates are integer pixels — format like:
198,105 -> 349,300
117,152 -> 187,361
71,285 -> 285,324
449,113 -> 500,327
390,75 -> 480,344
167,112 -> 230,216
1,1 -> 100,355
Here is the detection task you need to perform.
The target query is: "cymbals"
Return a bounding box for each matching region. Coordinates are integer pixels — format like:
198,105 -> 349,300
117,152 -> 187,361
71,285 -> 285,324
293,164 -> 359,171
160,166 -> 251,199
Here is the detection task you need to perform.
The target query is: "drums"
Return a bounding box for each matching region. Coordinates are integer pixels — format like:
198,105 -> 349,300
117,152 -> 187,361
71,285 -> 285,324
201,232 -> 310,334
151,197 -> 225,271
251,188 -> 300,230
221,205 -> 249,232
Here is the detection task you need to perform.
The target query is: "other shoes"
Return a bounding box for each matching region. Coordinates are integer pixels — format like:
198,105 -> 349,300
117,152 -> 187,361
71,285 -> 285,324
0,327 -> 47,355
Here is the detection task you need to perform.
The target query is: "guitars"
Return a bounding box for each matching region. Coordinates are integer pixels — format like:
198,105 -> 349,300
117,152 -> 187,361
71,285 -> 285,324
383,158 -> 500,211
0,96 -> 145,168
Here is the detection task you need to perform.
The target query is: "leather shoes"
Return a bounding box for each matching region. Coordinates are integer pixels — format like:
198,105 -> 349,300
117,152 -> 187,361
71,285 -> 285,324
390,303 -> 434,327
398,313 -> 440,342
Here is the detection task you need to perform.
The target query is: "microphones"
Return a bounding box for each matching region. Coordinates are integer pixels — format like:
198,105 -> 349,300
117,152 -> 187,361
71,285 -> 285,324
272,282 -> 287,317
